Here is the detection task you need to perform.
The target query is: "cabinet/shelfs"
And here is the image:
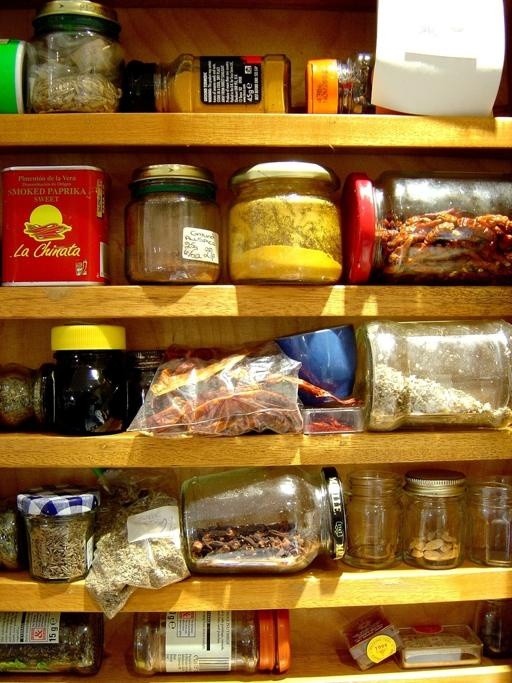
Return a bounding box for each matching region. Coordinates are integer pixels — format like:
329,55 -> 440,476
0,112 -> 512,683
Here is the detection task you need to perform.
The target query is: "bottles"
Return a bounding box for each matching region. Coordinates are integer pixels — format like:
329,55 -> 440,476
341,469 -> 405,567
29,0 -> 123,114
471,470 -> 511,568
359,318 -> 512,432
475,599 -> 512,659
15,482 -> 100,584
0,324 -> 128,436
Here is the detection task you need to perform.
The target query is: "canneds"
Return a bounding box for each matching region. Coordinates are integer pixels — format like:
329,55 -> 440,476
51,323 -> 137,435
26,0 -> 291,114
0,612 -> 104,675
132,610 -> 291,676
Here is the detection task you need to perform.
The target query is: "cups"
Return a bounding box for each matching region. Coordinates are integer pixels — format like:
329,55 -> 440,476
272,322 -> 358,398
